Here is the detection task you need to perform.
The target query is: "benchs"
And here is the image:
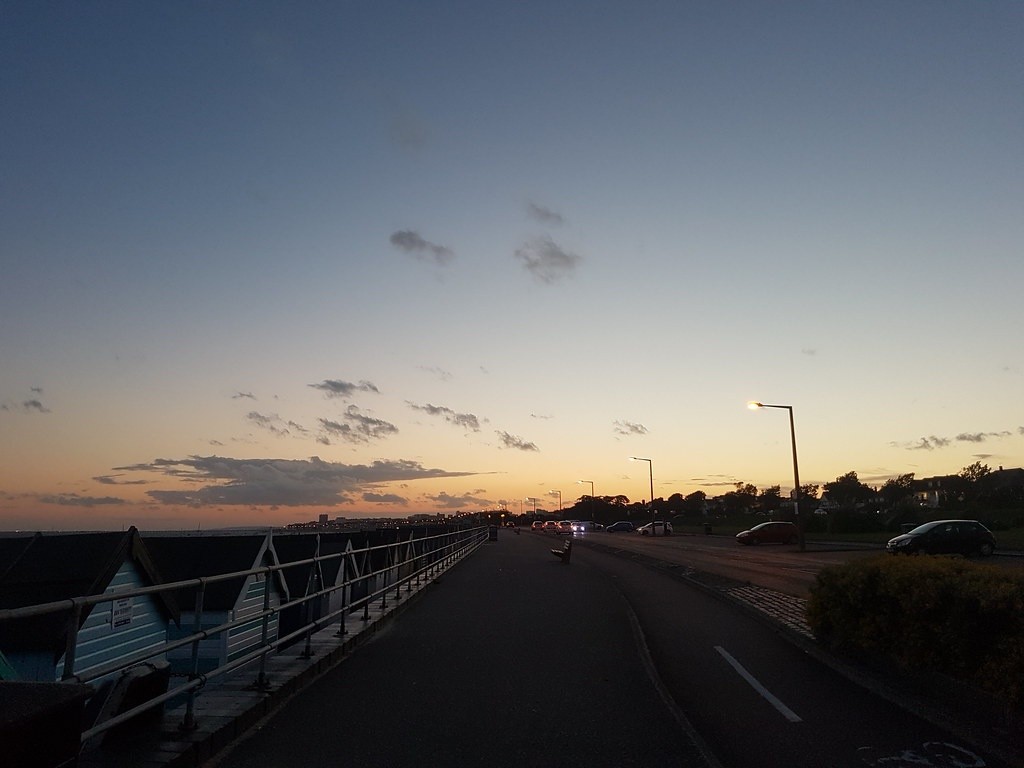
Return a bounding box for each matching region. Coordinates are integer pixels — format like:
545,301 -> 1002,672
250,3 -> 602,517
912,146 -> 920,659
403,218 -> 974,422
514,528 -> 521,535
550,539 -> 572,565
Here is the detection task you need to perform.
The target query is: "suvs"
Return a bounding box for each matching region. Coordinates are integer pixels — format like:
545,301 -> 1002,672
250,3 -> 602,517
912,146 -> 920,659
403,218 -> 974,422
606,521 -> 634,533
636,521 -> 674,538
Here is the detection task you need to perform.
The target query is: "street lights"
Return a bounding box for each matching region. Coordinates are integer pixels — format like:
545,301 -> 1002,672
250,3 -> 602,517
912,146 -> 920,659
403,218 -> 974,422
746,401 -> 806,549
627,456 -> 656,535
525,497 -> 536,521
549,489 -> 561,520
579,480 -> 596,532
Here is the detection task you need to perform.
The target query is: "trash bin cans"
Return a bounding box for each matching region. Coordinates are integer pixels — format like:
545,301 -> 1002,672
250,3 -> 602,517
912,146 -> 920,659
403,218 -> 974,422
704,523 -> 712,534
901,523 -> 920,535
489,526 -> 497,541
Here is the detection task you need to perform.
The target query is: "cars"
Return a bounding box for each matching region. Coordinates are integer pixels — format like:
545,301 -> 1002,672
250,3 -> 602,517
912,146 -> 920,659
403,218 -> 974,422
506,521 -> 515,528
735,521 -> 798,545
884,518 -> 999,559
530,518 -> 603,536
